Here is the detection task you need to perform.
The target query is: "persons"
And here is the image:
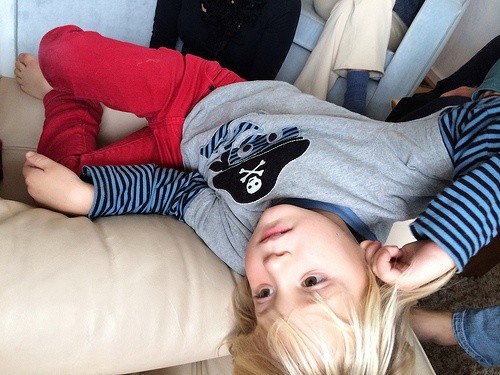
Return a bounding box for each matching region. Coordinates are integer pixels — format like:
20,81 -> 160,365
383,31 -> 500,124
409,306 -> 499,367
293,0 -> 424,118
14,23 -> 499,375
149,0 -> 302,83
